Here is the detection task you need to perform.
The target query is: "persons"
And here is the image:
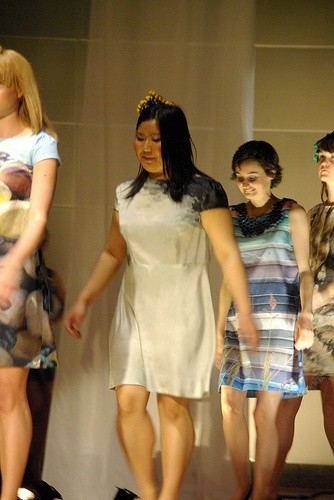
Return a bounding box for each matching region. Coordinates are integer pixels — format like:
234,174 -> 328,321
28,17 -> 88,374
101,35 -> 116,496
0,46 -> 62,500
64,95 -> 257,500
215,141 -> 314,500
272,132 -> 334,486
